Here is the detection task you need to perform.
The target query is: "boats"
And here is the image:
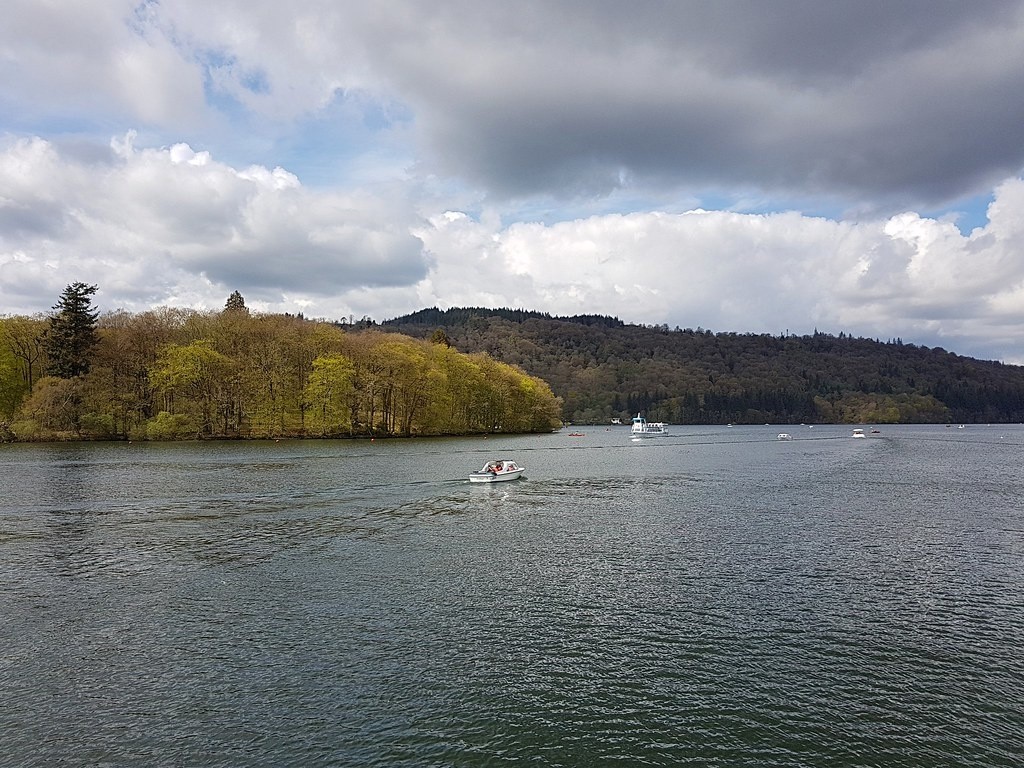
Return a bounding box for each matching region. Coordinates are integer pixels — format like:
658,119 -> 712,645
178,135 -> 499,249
631,412 -> 669,438
569,431 -> 586,436
777,433 -> 793,442
610,417 -> 630,426
851,428 -> 867,439
468,460 -> 526,483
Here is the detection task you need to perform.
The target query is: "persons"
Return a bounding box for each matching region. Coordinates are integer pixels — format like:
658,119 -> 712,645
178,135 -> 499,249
509,464 -> 513,470
496,464 -> 502,471
